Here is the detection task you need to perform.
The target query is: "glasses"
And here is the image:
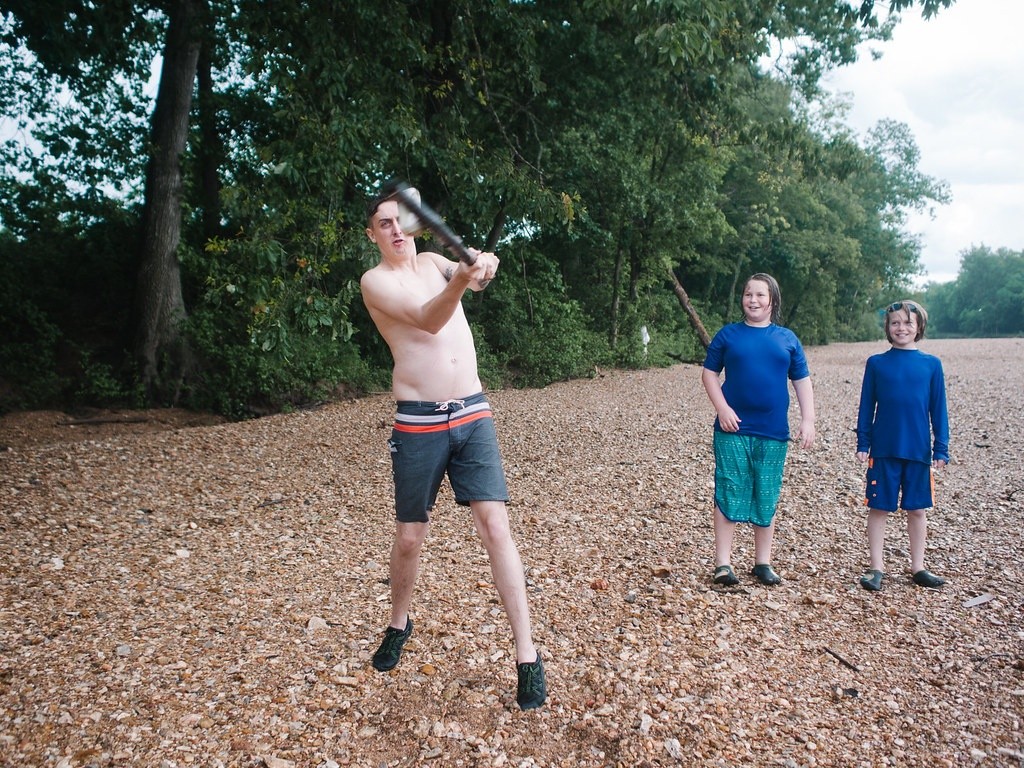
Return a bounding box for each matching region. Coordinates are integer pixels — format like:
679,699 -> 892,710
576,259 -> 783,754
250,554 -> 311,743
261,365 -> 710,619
889,303 -> 917,314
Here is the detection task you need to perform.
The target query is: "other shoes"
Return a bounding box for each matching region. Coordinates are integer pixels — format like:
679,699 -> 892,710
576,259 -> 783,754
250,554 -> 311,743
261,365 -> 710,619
913,569 -> 947,586
752,563 -> 782,585
860,571 -> 883,590
714,565 -> 739,586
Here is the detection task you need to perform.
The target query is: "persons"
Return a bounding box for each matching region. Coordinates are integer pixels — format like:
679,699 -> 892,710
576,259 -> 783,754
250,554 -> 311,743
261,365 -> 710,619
858,301 -> 948,591
360,193 -> 547,711
701,273 -> 815,585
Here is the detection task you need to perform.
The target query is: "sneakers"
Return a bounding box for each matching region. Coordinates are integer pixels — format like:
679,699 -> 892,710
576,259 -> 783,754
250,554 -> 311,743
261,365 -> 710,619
371,613 -> 412,672
515,652 -> 546,710
